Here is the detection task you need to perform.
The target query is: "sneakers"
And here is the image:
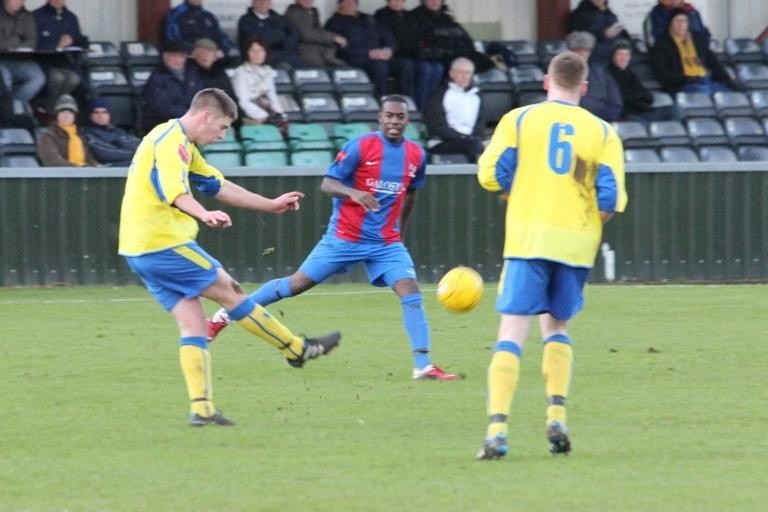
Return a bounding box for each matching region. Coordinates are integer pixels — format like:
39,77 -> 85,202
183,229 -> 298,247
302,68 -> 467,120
546,421 -> 572,456
285,330 -> 342,369
187,410 -> 235,428
202,308 -> 231,342
412,365 -> 463,384
475,433 -> 509,460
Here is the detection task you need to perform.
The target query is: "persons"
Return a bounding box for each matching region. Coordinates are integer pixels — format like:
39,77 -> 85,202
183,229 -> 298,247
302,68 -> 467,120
1,0 -> 142,165
208,96 -> 466,382
237,0 -> 395,100
147,0 -> 287,136
118,88 -> 341,426
374,1 -> 493,165
476,52 -> 629,460
566,0 -> 739,121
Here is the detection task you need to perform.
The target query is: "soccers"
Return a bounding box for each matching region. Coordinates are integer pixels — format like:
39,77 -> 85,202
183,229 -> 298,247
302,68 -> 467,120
436,266 -> 484,312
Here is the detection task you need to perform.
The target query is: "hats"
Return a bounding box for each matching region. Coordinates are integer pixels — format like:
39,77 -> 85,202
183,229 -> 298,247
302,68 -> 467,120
605,39 -> 632,63
160,37 -> 219,52
565,30 -> 597,51
87,96 -> 114,113
665,6 -> 688,26
52,93 -> 80,115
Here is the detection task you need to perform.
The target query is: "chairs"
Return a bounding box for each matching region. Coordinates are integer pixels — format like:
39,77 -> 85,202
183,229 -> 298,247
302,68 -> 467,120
1,32 -> 768,163
